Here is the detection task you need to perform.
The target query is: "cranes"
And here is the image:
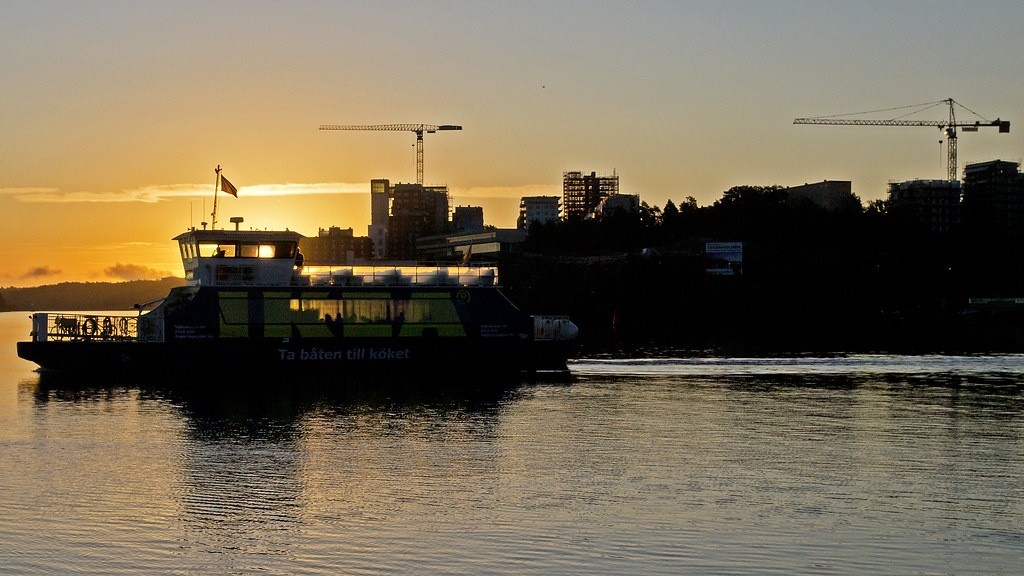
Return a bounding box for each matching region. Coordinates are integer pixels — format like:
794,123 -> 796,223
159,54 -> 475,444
318,123 -> 463,186
793,97 -> 1010,181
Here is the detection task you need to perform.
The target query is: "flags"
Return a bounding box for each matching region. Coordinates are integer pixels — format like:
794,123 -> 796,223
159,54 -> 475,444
221,175 -> 238,197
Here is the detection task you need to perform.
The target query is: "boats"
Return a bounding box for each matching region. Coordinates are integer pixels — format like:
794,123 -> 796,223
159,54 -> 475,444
135,165 -> 536,389
